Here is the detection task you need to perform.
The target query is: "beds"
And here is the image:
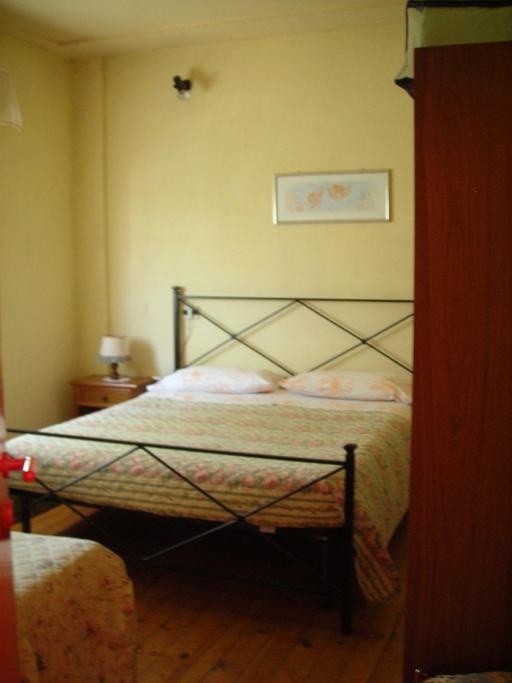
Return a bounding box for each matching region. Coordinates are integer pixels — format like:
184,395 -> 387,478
5,287 -> 412,637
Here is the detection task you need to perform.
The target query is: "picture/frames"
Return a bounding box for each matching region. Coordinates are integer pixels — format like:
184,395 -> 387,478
271,169 -> 393,222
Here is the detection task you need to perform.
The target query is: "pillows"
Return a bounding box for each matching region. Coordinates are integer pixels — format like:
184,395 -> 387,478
146,365 -> 412,402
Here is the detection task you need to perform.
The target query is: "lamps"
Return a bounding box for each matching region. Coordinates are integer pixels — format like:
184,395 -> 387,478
96,336 -> 130,379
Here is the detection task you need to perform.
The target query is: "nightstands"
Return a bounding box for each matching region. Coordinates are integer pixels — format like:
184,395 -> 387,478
68,375 -> 155,417
